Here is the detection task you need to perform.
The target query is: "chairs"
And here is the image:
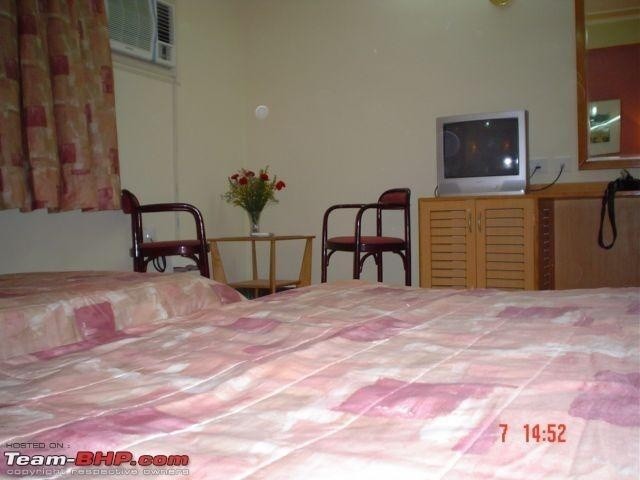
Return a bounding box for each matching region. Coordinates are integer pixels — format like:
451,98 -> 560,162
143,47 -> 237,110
321,187 -> 412,288
120,187 -> 210,278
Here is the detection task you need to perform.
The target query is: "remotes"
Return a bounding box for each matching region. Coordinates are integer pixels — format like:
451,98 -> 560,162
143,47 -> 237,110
251,232 -> 274,237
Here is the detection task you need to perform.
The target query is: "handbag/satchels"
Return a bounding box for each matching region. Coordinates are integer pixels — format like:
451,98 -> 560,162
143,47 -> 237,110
611,178 -> 640,191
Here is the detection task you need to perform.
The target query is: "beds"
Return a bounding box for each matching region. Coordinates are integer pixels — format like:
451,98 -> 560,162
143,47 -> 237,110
2,273 -> 640,480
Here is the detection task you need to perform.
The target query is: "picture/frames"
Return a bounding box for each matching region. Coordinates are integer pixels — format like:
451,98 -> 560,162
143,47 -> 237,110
587,98 -> 621,157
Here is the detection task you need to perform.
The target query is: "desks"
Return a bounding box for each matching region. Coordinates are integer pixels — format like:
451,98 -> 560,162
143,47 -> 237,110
204,235 -> 317,299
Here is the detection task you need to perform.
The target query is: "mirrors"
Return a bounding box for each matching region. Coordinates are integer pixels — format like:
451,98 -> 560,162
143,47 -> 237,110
574,1 -> 639,170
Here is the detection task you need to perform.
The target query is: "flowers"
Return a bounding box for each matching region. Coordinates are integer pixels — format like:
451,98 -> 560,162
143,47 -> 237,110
218,164 -> 287,226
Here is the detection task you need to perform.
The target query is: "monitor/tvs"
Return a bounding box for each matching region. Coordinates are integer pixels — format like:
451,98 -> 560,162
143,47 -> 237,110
436,110 -> 528,197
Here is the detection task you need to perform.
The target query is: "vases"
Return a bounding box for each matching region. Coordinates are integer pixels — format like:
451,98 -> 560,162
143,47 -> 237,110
249,210 -> 259,235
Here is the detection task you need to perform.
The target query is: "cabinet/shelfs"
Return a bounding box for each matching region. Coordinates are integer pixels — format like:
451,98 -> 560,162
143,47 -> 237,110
418,195 -> 537,290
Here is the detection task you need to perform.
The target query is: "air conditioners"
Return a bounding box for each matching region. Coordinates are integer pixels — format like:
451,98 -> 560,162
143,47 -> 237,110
105,0 -> 176,69
